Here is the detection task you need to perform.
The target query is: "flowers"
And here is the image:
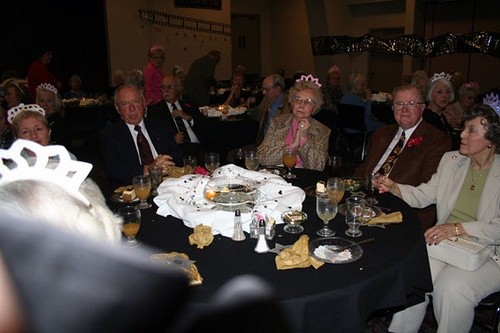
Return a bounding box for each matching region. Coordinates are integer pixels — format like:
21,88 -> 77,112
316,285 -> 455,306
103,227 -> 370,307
407,136 -> 423,153
195,166 -> 209,176
342,178 -> 360,191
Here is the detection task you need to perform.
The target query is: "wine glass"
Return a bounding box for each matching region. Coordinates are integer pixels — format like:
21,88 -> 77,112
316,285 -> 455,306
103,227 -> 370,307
280,210 -> 307,234
204,176 -> 258,215
282,146 -> 298,179
326,178 -> 345,215
316,193 -> 339,238
132,175 -> 153,208
345,197 -> 365,237
148,166 -> 163,196
116,206 -> 142,248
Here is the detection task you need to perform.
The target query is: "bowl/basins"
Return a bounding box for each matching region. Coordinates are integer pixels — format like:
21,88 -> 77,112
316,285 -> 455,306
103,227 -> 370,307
309,238 -> 364,264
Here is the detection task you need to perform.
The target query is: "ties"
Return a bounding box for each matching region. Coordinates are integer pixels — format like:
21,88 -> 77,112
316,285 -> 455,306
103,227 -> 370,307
373,129 -> 406,181
170,102 -> 191,145
134,125 -> 155,166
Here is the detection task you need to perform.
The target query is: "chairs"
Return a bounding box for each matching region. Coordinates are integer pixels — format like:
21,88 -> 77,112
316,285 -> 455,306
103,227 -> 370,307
336,103 -> 375,162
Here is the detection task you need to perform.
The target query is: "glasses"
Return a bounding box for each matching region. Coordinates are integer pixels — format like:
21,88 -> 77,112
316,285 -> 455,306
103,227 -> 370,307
293,96 -> 316,107
391,100 -> 426,111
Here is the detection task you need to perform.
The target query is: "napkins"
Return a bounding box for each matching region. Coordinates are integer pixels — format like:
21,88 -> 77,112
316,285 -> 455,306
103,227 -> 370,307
189,223 -> 214,249
149,252 -> 203,285
275,234 -> 325,270
367,211 -> 402,223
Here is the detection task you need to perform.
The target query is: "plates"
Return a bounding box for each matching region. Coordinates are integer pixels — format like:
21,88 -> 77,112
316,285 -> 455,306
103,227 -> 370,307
337,203 -> 382,219
336,176 -> 362,192
305,186 -> 332,196
112,192 -> 140,202
259,167 -> 287,176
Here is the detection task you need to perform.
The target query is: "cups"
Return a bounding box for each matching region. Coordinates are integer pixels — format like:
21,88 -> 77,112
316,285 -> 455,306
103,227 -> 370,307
183,155 -> 196,166
245,151 -> 262,170
204,152 -> 220,173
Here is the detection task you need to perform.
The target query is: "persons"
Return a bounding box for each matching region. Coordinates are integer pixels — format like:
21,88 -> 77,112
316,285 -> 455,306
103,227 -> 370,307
0,47 -> 87,147
0,139 -> 123,247
147,51 -> 290,160
322,65 -> 343,109
353,84 -> 448,227
376,94 -> 500,333
141,45 -> 166,105
100,84 -> 175,187
100,69 -> 145,120
4,103 -> 78,168
342,72 -> 392,132
257,74 -> 331,170
405,70 -> 500,152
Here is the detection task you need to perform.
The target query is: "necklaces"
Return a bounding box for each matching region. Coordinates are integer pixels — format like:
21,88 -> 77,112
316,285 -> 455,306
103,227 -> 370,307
470,158 -> 494,190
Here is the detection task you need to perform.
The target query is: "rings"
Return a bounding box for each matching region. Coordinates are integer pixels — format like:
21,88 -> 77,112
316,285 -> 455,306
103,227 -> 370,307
301,124 -> 304,127
433,235 -> 438,239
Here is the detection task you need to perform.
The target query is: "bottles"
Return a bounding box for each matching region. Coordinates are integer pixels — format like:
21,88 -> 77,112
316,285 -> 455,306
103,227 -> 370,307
232,209 -> 246,241
253,219 -> 271,254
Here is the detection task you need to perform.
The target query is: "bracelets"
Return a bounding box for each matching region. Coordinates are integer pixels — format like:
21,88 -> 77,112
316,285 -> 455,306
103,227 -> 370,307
454,223 -> 459,236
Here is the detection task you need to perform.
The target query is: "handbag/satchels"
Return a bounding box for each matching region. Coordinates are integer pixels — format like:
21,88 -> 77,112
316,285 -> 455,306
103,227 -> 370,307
426,234 -> 500,273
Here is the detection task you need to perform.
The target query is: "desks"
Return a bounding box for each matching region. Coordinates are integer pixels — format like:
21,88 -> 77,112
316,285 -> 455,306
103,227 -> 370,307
183,105 -> 259,153
61,104 -> 116,140
105,168 -> 420,333
